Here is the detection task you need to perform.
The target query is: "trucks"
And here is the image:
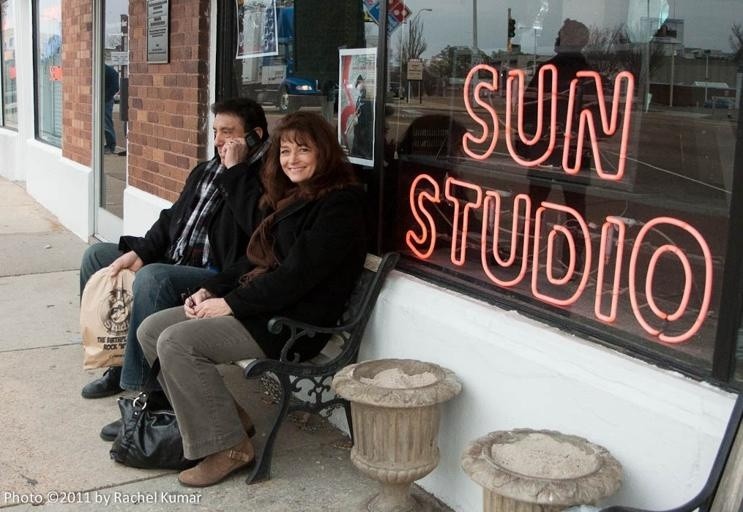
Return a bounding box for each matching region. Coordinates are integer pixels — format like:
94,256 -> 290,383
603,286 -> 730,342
235,4 -> 344,116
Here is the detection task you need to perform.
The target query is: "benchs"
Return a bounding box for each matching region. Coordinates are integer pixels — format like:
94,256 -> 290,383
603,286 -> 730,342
234,249 -> 404,485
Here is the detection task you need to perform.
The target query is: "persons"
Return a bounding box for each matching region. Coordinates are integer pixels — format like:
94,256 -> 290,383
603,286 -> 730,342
378,113 -> 491,250
133,110 -> 376,490
103,63 -> 121,152
515,17 -> 623,276
77,96 -> 274,443
352,73 -> 375,159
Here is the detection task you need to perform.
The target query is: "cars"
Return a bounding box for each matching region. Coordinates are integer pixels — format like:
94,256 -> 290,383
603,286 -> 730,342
371,98 -> 743,322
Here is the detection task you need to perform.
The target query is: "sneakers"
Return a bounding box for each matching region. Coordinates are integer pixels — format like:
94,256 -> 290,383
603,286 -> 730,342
104,143 -> 126,156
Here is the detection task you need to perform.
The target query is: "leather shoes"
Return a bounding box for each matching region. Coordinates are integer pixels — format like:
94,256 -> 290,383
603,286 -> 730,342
100,404 -> 171,441
178,406 -> 257,487
81,367 -> 126,398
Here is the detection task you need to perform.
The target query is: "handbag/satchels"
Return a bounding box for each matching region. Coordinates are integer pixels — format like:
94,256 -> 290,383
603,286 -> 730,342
109,393 -> 201,470
80,267 -> 136,371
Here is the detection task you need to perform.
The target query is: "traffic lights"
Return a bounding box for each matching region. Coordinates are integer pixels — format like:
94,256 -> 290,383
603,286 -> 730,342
508,18 -> 516,38
